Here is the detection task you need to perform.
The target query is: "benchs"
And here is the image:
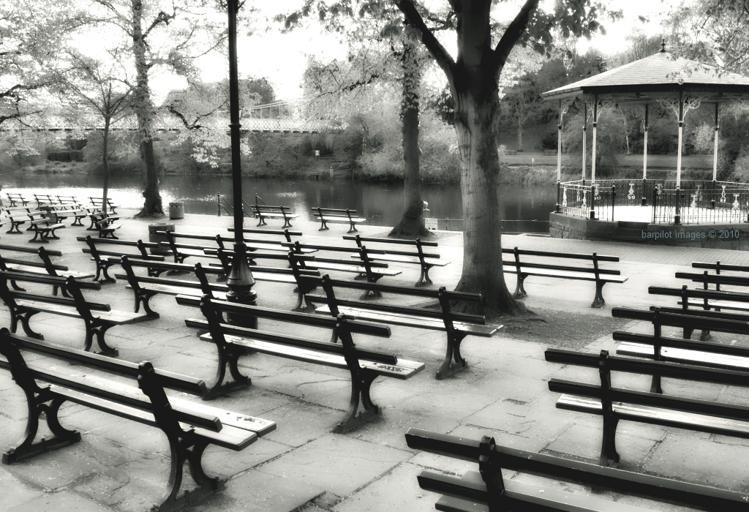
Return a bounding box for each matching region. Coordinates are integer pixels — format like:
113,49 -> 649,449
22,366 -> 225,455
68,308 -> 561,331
535,341 -> 749,470
293,270 -> 506,383
172,292 -> 429,435
686,257 -> 748,334
645,271 -> 748,348
605,302 -> 748,407
493,245 -> 632,311
641,280 -> 746,398
2,190 -> 453,362
0,324 -> 278,510
394,421 -> 749,511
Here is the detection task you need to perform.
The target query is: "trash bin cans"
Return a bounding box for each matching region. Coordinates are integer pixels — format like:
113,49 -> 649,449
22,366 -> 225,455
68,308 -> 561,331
169,202 -> 184,218
149,223 -> 175,255
